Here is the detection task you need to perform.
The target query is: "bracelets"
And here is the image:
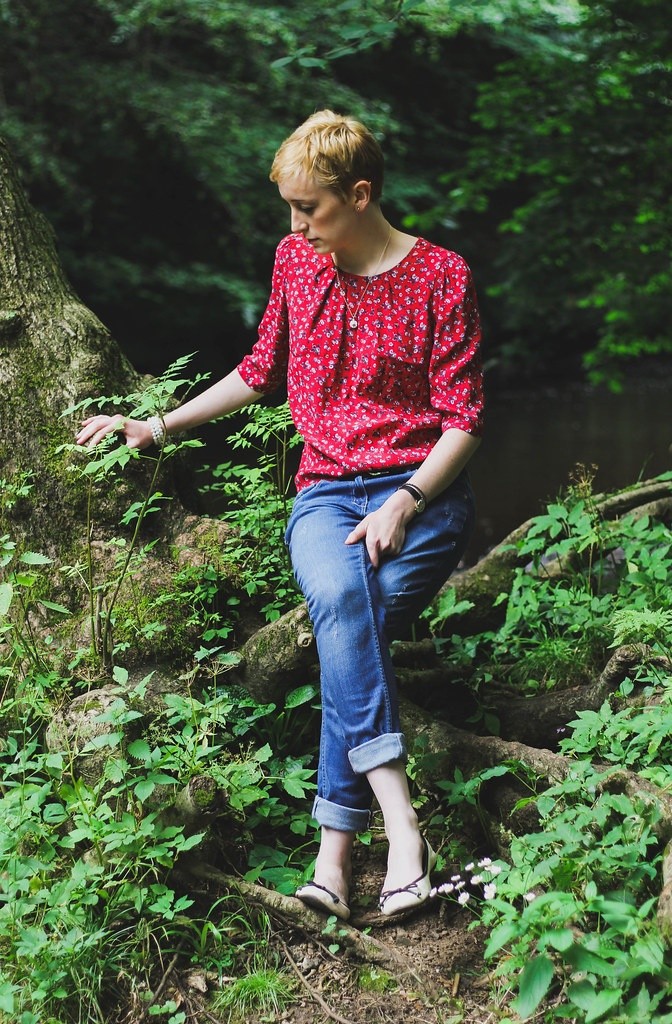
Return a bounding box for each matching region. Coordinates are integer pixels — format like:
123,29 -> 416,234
147,416 -> 164,446
404,483 -> 427,508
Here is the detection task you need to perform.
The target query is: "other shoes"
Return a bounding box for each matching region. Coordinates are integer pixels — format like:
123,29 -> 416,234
296,881 -> 350,920
378,830 -> 438,915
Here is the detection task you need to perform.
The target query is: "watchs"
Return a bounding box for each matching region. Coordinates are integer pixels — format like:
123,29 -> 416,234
395,485 -> 425,513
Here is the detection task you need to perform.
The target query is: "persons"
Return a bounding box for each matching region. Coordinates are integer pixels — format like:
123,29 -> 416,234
77,108 -> 488,919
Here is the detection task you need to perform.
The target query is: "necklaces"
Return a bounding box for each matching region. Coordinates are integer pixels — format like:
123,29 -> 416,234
333,225 -> 392,329
